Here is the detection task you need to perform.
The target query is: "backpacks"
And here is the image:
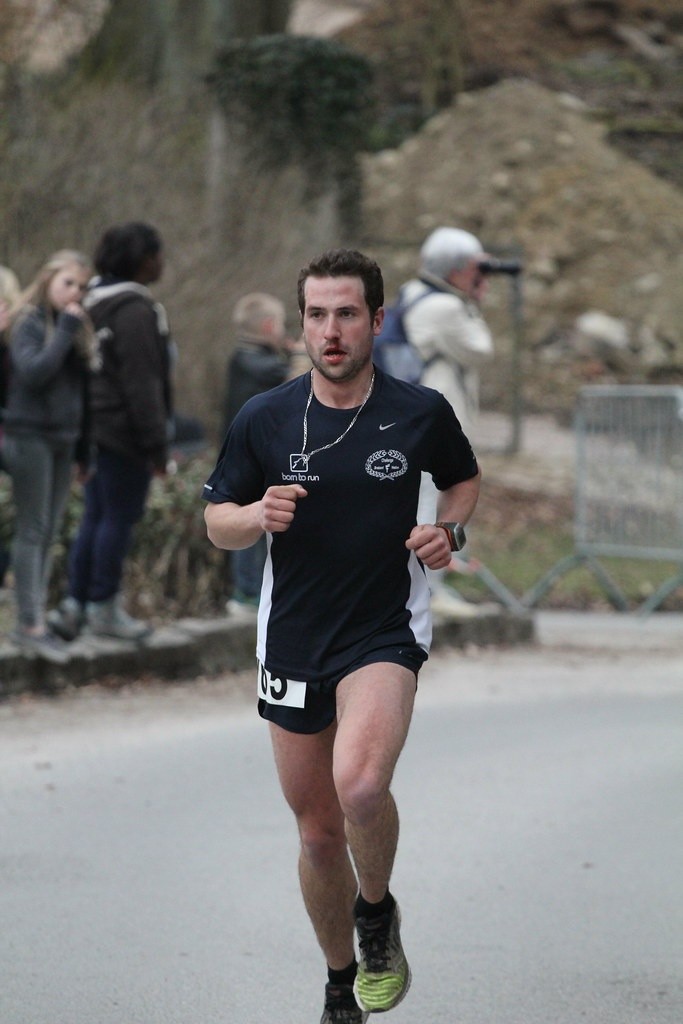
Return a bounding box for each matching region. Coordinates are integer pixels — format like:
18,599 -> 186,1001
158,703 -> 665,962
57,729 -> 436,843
370,283 -> 439,385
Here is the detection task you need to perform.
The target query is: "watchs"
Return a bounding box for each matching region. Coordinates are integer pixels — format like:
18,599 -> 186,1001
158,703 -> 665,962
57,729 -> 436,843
434,521 -> 466,552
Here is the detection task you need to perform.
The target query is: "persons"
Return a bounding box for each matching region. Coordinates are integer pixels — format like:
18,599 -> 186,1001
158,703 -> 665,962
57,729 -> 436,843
402,226 -> 495,654
0,223 -> 176,662
201,250 -> 480,1024
222,291 -> 297,614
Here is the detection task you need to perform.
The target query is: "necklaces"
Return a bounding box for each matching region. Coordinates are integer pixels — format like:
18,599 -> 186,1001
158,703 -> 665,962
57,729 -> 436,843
301,368 -> 375,463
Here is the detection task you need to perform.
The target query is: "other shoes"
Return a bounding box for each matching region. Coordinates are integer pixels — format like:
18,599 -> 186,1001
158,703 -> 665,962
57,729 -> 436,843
42,596 -> 86,641
225,600 -> 258,622
82,599 -> 154,638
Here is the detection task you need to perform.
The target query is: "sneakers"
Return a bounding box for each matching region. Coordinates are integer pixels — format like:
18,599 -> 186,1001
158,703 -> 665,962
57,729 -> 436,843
352,887 -> 413,1013
320,983 -> 370,1024
